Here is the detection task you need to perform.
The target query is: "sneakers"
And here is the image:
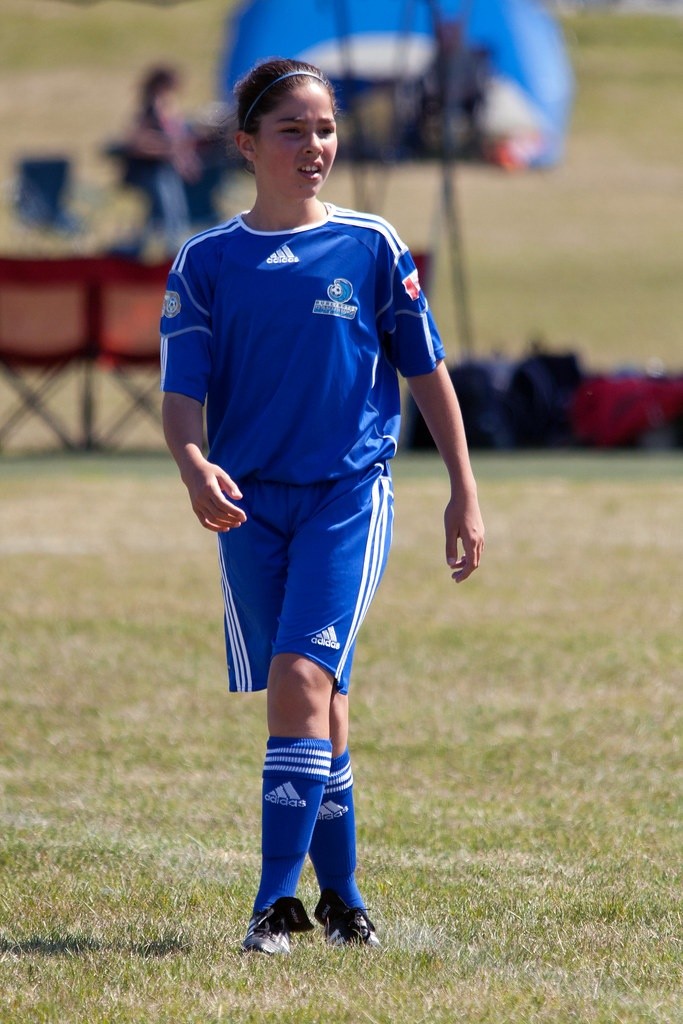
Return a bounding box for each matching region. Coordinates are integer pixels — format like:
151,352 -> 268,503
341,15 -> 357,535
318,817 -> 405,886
315,889 -> 383,950
243,897 -> 315,957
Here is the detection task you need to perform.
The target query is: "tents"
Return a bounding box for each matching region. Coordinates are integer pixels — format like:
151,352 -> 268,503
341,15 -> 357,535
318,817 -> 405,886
222,3 -> 571,170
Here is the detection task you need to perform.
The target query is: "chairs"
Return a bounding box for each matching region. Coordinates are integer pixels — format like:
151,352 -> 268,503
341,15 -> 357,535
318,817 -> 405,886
0,251 -> 180,465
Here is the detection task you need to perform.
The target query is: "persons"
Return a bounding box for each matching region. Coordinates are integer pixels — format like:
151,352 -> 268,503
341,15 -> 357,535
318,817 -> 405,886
127,58 -> 232,270
157,59 -> 485,955
